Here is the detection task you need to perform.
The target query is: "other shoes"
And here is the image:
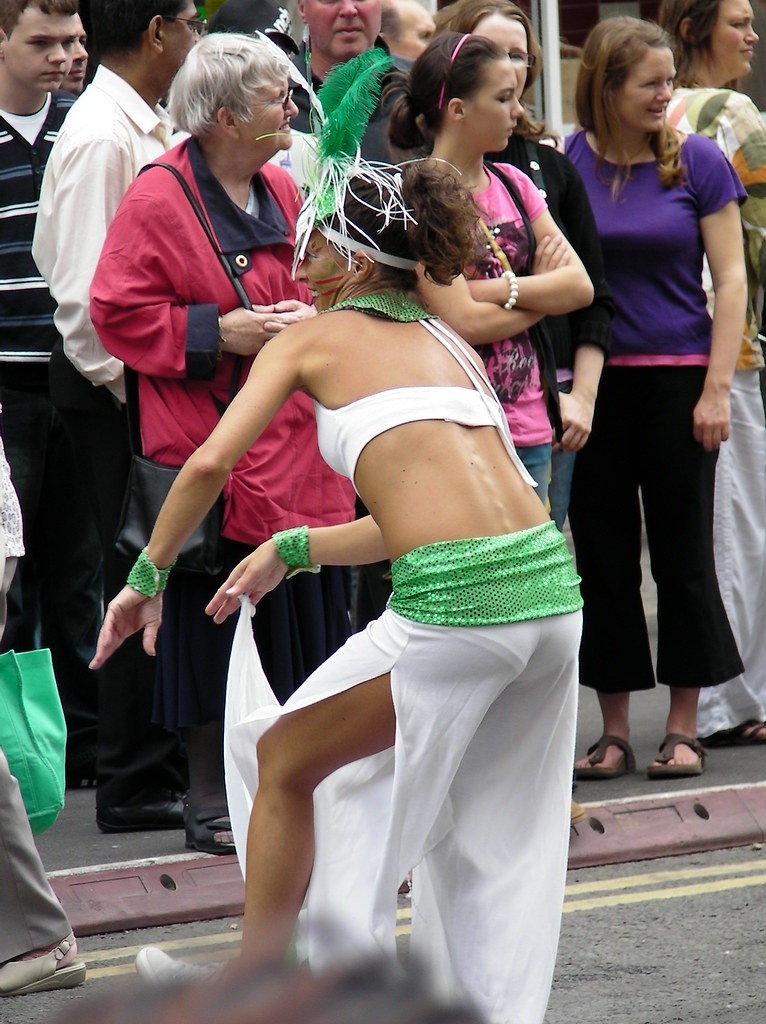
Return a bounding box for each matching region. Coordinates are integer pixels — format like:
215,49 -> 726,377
96,788 -> 188,833
134,946 -> 229,991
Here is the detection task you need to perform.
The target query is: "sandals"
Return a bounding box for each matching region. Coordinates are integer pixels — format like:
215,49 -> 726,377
185,807 -> 236,855
574,736 -> 636,779
698,719 -> 766,749
647,733 -> 707,777
0,932 -> 88,997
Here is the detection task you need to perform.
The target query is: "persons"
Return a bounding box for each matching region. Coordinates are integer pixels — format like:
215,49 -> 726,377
1,0 -> 766,1000
89,157 -> 584,1024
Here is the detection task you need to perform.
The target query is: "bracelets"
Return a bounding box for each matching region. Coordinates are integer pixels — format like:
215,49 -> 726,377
216,314 -> 228,360
270,525 -> 320,580
501,271 -> 518,309
126,549 -> 180,599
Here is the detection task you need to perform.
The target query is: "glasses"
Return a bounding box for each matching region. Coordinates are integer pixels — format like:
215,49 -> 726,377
161,14 -> 208,35
509,52 -> 536,68
247,89 -> 294,110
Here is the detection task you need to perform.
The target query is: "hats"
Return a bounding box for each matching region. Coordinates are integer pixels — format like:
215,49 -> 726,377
207,0 -> 301,55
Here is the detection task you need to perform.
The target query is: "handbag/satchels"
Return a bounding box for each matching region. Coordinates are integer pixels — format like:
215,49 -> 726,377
0,648 -> 68,838
111,455 -> 226,576
